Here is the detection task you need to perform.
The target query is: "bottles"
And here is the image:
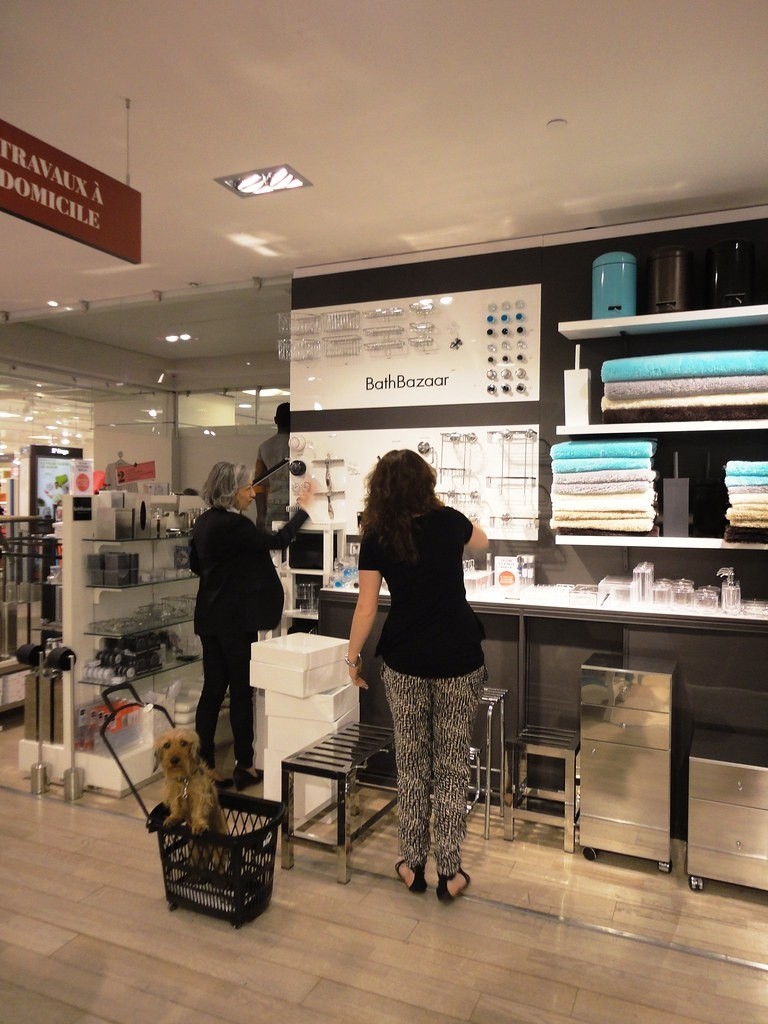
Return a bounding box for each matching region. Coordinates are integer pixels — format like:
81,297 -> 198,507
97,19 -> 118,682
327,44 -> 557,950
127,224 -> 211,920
335,566 -> 359,587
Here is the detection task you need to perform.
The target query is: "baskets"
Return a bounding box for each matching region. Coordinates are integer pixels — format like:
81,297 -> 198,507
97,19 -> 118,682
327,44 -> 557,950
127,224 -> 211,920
100,681 -> 288,926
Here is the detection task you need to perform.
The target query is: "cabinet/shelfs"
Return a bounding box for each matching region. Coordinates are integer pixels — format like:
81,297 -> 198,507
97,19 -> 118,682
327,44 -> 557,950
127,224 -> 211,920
19,493 -> 233,794
555,304 -> 768,550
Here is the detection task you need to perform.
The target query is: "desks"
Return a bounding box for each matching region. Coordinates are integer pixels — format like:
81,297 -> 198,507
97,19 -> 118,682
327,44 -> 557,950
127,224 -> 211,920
319,569 -> 768,818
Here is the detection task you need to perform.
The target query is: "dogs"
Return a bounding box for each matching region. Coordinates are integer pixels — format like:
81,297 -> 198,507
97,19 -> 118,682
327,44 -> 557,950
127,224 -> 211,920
151,725 -> 230,885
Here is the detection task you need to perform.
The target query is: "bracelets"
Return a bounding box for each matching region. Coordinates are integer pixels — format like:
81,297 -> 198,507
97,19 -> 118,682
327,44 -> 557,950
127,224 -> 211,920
345,653 -> 361,667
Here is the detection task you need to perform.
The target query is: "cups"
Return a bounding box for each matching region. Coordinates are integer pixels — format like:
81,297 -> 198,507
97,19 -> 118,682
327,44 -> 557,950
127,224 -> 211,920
632,562 -> 721,612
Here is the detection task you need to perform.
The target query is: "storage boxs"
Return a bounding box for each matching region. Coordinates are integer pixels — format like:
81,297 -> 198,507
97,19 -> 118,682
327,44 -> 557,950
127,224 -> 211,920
248,631 -> 361,823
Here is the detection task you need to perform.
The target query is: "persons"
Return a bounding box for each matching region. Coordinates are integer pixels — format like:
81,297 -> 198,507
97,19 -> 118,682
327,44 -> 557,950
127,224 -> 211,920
189,462 -> 312,791
347,449 -> 491,902
255,402 -> 290,531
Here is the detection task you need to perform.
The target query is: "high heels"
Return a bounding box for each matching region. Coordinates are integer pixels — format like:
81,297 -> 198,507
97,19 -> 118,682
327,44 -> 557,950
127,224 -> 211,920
233,765 -> 263,790
215,777 -> 233,788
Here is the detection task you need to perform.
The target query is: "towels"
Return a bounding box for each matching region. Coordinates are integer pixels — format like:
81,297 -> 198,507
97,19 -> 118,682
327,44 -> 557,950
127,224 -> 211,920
601,350 -> 768,425
724,461 -> 768,545
549,439 -> 660,536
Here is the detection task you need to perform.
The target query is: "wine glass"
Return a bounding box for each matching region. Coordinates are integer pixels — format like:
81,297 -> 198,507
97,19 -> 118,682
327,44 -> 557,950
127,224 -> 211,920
336,558 -> 345,576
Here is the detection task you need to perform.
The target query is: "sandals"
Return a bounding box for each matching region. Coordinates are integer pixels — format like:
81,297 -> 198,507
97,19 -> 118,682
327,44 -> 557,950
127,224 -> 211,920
436,868 -> 472,902
395,859 -> 428,893
